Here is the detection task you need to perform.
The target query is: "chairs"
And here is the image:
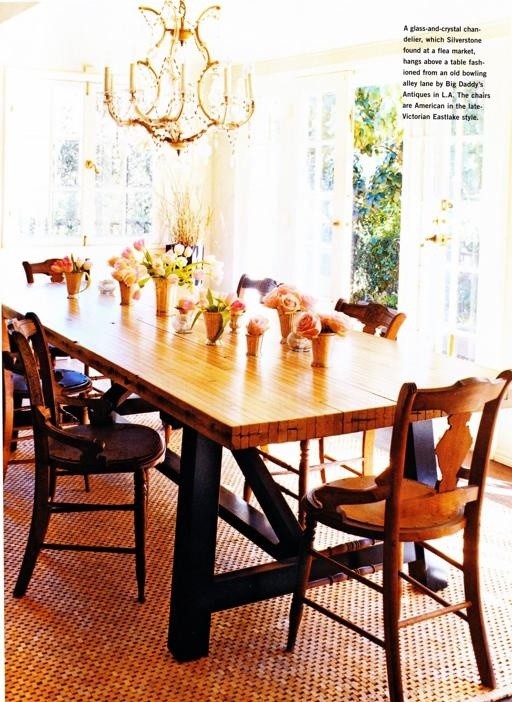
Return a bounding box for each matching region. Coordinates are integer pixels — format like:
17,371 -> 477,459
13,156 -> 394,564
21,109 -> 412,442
10,310 -> 166,605
4,306 -> 95,493
243,294 -> 404,530
284,367 -> 512,700
163,273 -> 288,466
22,259 -> 71,282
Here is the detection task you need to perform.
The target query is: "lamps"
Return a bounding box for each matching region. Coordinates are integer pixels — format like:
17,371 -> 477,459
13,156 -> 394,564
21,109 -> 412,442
82,0 -> 258,156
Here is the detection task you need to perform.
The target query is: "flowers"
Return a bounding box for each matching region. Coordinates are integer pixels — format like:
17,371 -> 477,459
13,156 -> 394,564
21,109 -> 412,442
50,239 -> 348,371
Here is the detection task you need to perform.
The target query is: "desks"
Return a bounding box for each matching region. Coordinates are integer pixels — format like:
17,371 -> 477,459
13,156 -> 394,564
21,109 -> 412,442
5,281 -> 511,667
